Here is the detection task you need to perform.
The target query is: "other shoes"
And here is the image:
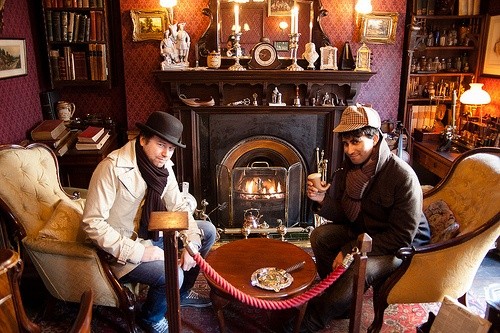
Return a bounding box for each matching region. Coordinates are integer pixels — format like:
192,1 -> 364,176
280,312 -> 324,333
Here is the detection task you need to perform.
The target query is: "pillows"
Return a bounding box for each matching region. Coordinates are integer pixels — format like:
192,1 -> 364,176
423,199 -> 460,244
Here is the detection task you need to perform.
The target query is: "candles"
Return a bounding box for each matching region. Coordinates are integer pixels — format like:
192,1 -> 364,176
294,6 -> 299,34
234,5 -> 239,33
291,9 -> 294,34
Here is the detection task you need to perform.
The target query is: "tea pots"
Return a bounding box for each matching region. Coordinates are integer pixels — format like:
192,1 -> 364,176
243,208 -> 263,228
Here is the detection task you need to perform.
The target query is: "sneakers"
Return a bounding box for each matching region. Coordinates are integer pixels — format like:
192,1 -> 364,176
136,317 -> 168,333
180,290 -> 212,307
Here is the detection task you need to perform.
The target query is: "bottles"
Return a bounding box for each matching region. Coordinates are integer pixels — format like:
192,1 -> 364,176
411,25 -> 470,98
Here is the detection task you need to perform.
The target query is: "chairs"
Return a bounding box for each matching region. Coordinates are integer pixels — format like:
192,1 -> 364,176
366,146 -> 500,333
0,142 -> 139,333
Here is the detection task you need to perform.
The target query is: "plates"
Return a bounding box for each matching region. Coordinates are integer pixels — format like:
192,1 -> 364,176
251,267 -> 293,290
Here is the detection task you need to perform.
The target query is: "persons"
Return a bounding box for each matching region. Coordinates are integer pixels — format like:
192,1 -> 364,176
82,112 -> 216,333
160,23 -> 190,63
284,102 -> 430,333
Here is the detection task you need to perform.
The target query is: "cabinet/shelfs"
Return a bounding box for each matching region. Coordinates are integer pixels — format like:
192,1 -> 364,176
41,0 -> 112,90
398,0 -> 484,142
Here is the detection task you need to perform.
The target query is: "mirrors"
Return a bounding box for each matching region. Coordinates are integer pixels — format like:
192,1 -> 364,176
217,0 -> 313,60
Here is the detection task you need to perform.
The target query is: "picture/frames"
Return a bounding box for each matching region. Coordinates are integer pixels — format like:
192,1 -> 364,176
478,13 -> 500,79
320,45 -> 339,70
268,0 -> 294,17
359,11 -> 399,45
130,8 -> 168,42
0,37 -> 28,80
273,40 -> 289,52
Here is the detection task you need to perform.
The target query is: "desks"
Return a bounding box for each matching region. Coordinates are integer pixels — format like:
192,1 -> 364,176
203,238 -> 317,333
407,117 -> 500,181
58,115 -> 119,189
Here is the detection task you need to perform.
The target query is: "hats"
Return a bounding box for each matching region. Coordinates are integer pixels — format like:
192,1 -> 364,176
135,111 -> 186,149
333,102 -> 381,132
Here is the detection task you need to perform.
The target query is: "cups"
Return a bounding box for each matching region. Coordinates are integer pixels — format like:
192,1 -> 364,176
207,51 -> 221,69
56,101 -> 75,121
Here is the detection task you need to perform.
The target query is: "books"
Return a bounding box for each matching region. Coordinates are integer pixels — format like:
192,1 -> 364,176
44,0 -> 109,82
415,0 -> 481,16
410,104 -> 436,132
30,120 -> 111,157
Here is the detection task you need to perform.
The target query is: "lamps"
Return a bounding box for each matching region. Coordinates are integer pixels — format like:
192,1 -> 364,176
354,43 -> 372,72
460,82 -> 492,139
